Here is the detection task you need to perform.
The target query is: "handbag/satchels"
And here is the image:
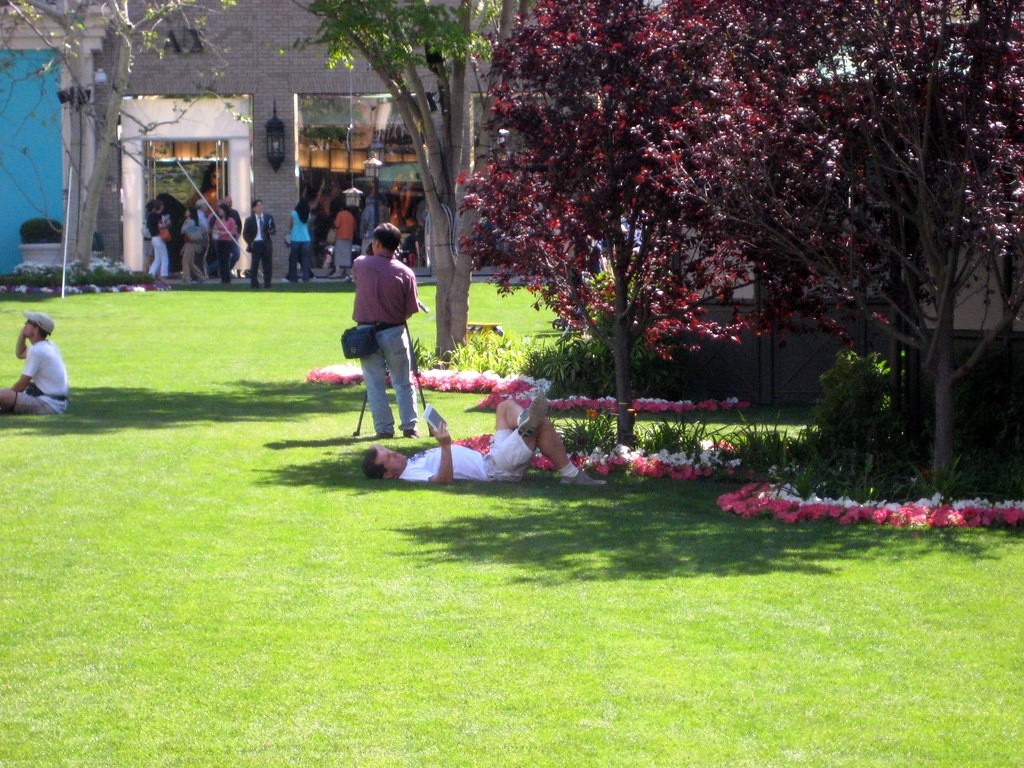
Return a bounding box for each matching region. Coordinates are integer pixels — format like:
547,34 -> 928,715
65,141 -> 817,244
185,225 -> 206,243
26,382 -> 67,401
160,228 -> 172,242
341,324 -> 402,359
326,228 -> 336,245
284,233 -> 291,245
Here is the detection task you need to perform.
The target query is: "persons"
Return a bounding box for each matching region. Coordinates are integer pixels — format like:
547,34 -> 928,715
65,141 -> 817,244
147,197 -> 391,290
360,393 -> 607,487
0,311 -> 69,415
352,222 -> 420,438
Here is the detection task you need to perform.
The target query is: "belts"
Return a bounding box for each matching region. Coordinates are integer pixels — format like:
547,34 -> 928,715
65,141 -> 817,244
358,322 -> 375,325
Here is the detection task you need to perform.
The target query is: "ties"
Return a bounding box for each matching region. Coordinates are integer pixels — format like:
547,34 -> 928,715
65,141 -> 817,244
258,216 -> 266,241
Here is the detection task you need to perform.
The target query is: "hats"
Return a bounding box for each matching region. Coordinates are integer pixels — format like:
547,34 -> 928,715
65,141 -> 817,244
196,199 -> 209,208
24,310 -> 54,336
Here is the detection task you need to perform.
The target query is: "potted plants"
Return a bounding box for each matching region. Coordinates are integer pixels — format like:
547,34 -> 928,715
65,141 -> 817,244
19,217 -> 63,265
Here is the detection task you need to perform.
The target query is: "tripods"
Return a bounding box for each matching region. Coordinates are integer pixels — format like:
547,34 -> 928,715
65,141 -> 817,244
351,302 -> 434,436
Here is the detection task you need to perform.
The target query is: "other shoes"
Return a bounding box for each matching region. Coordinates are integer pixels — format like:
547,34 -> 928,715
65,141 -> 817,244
376,433 -> 393,438
403,429 -> 420,438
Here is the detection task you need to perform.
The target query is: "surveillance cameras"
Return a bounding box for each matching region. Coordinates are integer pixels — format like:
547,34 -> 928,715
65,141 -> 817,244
95,73 -> 107,83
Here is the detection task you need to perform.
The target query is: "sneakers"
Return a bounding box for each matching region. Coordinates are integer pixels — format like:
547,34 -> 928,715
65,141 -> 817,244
518,395 -> 548,439
560,467 -> 607,485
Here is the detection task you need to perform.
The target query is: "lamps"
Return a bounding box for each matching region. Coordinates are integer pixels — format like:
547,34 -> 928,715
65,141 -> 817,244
343,103 -> 384,207
264,95 -> 285,172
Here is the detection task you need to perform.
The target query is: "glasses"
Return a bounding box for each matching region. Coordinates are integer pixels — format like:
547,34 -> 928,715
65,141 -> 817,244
28,320 -> 36,328
